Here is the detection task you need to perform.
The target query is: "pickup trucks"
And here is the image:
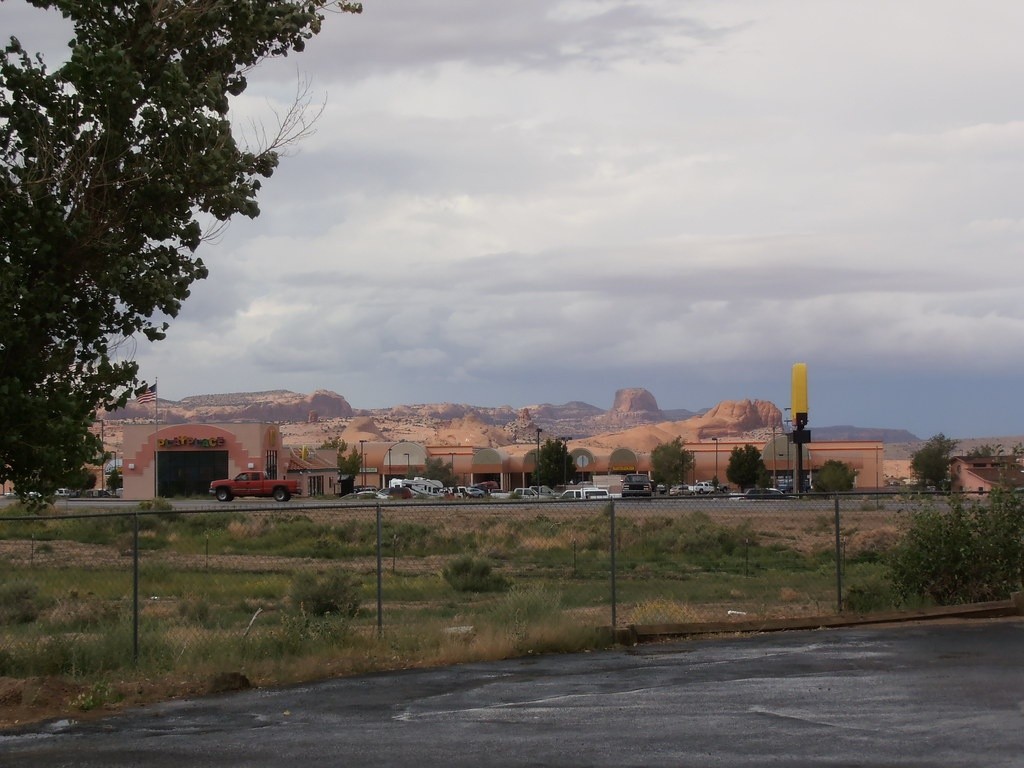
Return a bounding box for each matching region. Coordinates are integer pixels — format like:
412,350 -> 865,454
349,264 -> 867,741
688,480 -> 727,494
491,486 -> 622,499
209,471 -> 302,502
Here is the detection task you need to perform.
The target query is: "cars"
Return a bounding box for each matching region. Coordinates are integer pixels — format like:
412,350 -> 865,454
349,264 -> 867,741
340,485 -> 414,500
24,489 -> 123,498
657,484 -> 689,496
729,477 -> 807,500
439,481 -> 499,499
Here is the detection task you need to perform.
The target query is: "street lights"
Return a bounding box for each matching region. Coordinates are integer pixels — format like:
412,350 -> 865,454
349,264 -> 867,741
712,438 -> 718,477
95,419 -> 104,498
449,453 -> 456,477
535,428 -> 543,499
561,436 -> 573,491
387,448 -> 392,478
404,453 -> 409,478
359,440 -> 368,491
110,451 -> 116,496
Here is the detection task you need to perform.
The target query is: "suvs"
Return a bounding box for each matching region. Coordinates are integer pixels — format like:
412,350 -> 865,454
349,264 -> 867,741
620,474 -> 654,497
